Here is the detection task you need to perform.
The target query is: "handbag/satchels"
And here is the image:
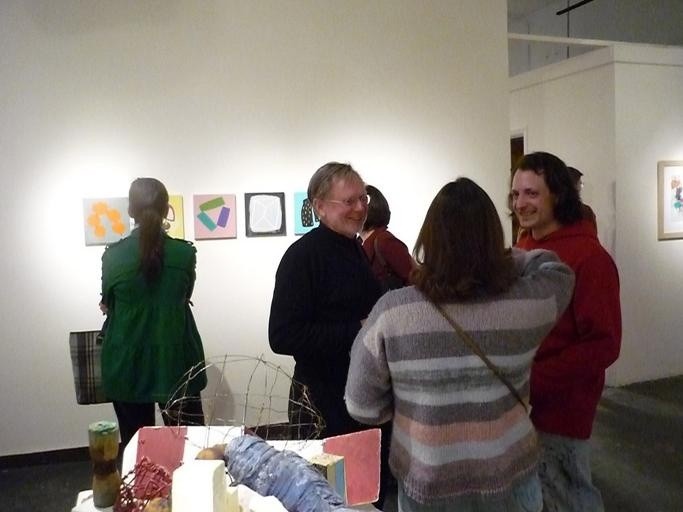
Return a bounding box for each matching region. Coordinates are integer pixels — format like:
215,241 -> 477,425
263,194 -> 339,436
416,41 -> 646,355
68,330 -> 113,405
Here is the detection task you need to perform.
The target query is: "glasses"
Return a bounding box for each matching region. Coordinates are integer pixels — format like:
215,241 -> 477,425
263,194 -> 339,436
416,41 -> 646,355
322,195 -> 368,206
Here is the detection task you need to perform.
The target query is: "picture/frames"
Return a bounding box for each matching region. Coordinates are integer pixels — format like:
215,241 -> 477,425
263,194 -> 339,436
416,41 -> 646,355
656,159 -> 682,240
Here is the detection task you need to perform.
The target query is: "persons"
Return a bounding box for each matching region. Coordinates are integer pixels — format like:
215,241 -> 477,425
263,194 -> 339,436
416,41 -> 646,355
360,184 -> 419,288
343,175 -> 578,512
510,150 -> 623,512
96,177 -> 209,467
265,159 -> 394,512
568,165 -> 584,196
